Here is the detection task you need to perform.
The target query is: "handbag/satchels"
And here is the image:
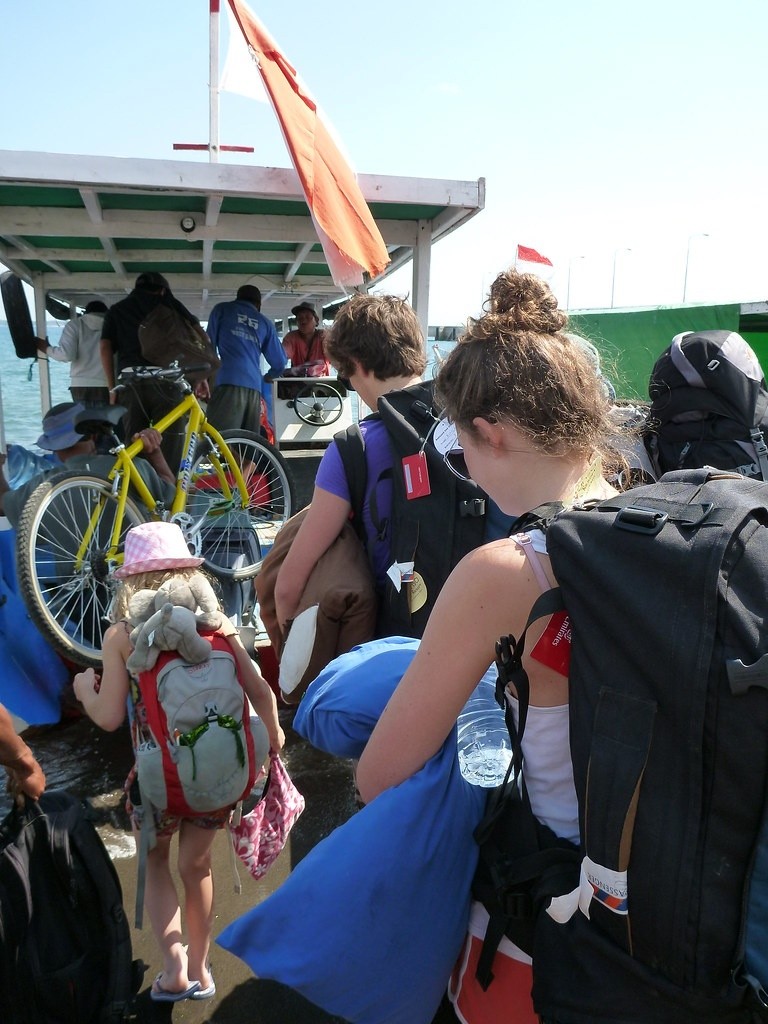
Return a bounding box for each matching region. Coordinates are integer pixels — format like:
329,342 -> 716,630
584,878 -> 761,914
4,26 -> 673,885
230,744 -> 307,891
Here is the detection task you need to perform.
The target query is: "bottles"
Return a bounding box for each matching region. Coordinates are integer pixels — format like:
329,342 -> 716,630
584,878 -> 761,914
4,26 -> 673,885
454,661 -> 522,788
7,444 -> 56,490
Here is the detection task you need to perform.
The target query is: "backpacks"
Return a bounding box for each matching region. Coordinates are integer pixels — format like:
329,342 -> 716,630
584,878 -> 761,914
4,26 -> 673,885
136,630 -> 257,818
0,791 -> 145,1024
476,470 -> 768,1024
640,328 -> 768,474
161,293 -> 221,381
331,381 -> 491,643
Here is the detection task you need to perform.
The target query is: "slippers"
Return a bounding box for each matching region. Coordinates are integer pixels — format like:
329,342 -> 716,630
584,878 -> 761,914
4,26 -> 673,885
186,973 -> 216,1000
150,971 -> 202,1002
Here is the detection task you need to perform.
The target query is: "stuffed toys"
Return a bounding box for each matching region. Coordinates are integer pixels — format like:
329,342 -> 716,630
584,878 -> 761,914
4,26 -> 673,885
125,568 -> 229,676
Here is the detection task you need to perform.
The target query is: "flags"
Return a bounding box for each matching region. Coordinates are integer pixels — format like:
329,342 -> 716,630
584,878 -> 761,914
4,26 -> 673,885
229,0 -> 392,290
517,241 -> 554,264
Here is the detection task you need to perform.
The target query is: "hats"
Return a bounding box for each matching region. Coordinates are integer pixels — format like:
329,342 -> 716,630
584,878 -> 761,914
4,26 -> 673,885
291,301 -> 318,316
135,272 -> 171,290
36,402 -> 91,451
114,521 -> 206,581
238,285 -> 261,302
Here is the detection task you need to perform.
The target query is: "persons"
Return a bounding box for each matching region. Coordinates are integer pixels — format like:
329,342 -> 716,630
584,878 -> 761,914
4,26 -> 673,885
100,269 -> 210,464
0,703 -> 47,813
0,400 -> 179,646
72,520 -> 286,1003
208,283 -> 288,458
282,301 -> 330,379
353,268 -> 625,1024
31,297 -> 116,410
274,293 -> 527,641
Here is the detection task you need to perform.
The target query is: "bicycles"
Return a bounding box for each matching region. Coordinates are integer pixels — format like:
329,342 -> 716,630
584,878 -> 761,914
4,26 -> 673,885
18,359 -> 296,670
